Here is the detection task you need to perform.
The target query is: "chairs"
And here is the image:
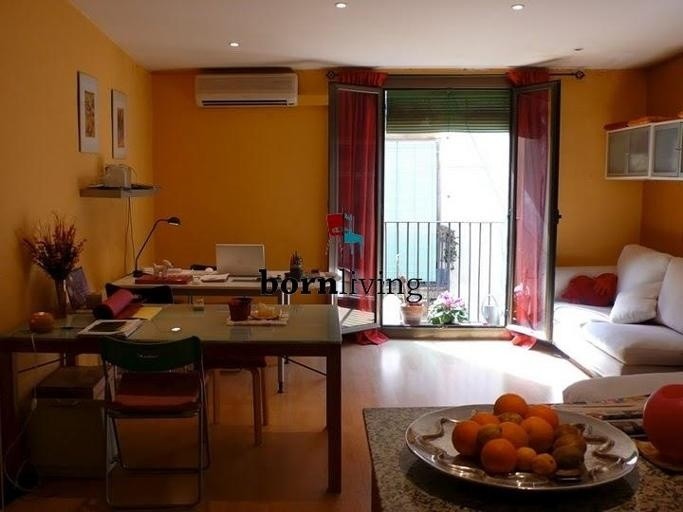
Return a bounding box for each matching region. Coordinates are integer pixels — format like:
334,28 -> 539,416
187,263 -> 217,305
209,357 -> 268,445
106,284 -> 173,304
99,334 -> 210,509
316,271 -> 382,346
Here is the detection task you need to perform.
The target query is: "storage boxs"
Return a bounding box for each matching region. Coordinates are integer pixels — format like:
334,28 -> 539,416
16,397 -> 111,480
36,365 -> 115,401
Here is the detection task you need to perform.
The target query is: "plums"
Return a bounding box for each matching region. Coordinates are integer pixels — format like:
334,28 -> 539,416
449,393 -> 586,475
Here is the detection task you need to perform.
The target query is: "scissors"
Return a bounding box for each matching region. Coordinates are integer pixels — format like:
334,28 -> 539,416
295,257 -> 303,265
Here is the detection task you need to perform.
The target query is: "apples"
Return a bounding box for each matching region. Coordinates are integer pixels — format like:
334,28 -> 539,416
640,381 -> 683,463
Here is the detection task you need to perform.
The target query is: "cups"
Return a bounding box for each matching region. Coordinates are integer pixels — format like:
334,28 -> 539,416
228,297 -> 252,322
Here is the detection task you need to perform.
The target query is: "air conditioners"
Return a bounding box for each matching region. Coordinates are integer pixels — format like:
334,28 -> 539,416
195,74 -> 298,107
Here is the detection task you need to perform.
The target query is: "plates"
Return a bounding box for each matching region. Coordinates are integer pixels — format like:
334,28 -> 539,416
405,404 -> 640,490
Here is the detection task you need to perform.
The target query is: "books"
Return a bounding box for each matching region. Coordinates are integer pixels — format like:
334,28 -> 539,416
201,273 -> 230,282
77,318 -> 143,340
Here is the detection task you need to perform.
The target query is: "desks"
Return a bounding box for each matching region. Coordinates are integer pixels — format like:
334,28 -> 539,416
105,268 -> 337,392
0,303 -> 343,494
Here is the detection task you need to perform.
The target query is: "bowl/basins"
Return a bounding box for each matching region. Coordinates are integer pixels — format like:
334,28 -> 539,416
251,303 -> 281,320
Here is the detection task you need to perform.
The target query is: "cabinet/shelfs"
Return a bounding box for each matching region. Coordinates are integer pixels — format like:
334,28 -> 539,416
648,119 -> 683,180
605,123 -> 653,181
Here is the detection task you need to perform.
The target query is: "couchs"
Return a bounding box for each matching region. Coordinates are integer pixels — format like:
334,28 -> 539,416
552,245 -> 683,377
562,371 -> 683,404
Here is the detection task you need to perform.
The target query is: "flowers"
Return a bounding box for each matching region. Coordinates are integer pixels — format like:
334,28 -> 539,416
427,291 -> 467,324
23,216 -> 87,280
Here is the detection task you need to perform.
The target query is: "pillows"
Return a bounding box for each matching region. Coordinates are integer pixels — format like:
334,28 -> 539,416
560,272 -> 619,307
611,255 -> 672,323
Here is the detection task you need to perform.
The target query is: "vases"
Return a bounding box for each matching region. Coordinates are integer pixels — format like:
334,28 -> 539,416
400,303 -> 424,324
55,280 -> 64,304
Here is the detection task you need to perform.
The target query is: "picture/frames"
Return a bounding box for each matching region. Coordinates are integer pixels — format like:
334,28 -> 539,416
77,69 -> 98,153
110,88 -> 128,159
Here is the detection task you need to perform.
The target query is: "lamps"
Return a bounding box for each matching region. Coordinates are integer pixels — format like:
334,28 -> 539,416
133,216 -> 182,277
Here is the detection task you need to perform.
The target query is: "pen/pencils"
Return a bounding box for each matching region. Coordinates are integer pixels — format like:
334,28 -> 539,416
291,251 -> 297,265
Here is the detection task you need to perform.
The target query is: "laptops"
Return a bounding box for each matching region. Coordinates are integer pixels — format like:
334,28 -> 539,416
215,243 -> 266,277
